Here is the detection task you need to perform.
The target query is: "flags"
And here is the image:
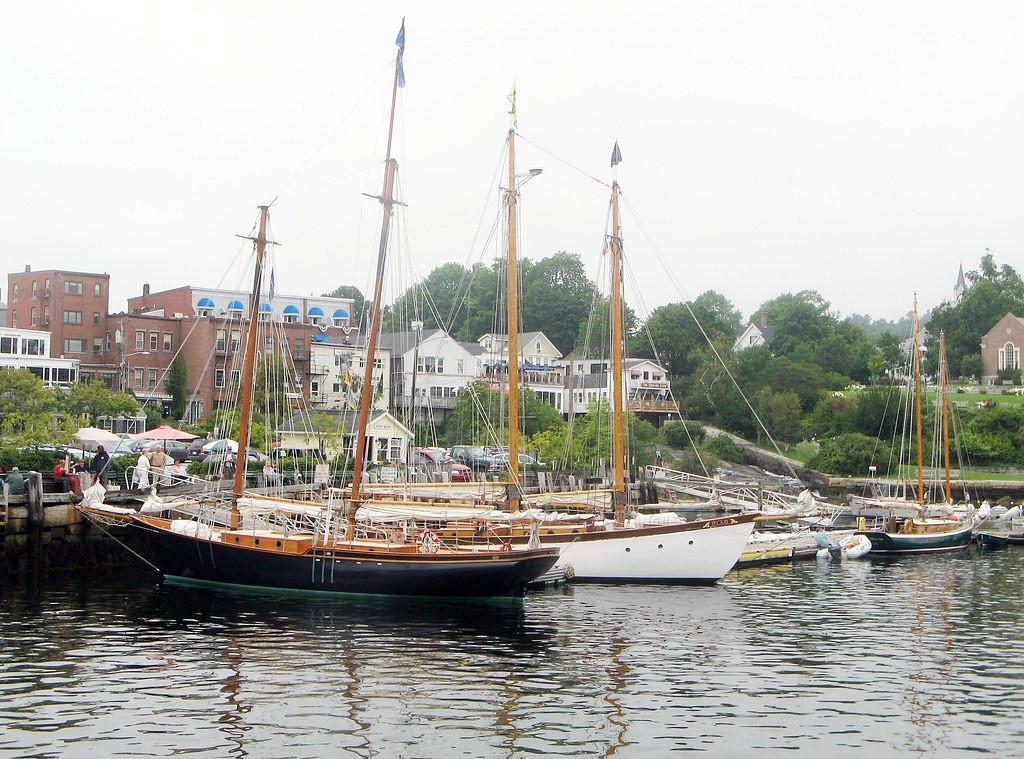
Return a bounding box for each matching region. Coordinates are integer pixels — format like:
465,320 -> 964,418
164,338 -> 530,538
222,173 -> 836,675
508,80 -> 519,131
396,23 -> 405,85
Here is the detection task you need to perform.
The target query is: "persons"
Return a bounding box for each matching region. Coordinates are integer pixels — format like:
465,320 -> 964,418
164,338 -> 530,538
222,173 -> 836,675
814,526 -> 830,547
0,432 -> 283,495
436,450 -> 451,482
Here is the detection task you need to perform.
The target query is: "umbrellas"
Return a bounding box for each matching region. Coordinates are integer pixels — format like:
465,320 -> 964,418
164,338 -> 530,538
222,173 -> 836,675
76,424 -> 239,468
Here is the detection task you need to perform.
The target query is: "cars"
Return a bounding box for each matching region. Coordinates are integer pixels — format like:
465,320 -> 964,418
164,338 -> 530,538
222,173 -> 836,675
410,448 -> 473,484
445,444 -> 497,472
131,439 -> 191,466
488,453 -> 547,468
186,438 -> 259,462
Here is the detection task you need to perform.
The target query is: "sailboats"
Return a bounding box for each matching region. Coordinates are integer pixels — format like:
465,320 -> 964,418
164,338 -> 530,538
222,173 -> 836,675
57,11 -> 1024,608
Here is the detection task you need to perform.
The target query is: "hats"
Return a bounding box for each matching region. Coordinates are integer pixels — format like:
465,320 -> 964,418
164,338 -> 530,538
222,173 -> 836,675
227,446 -> 232,450
